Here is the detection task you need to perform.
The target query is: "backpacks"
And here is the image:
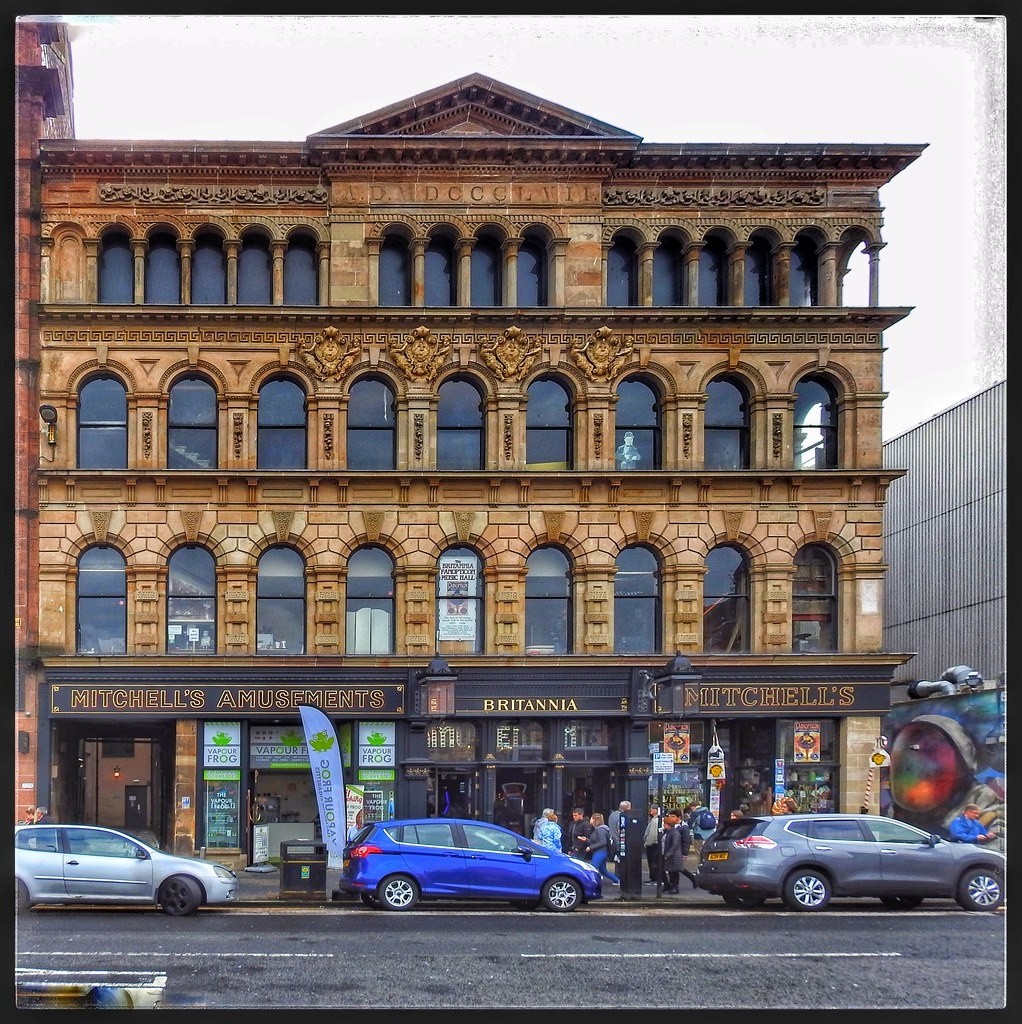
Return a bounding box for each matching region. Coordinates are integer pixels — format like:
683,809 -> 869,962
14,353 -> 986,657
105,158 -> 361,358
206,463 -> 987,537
608,832 -> 616,855
699,811 -> 716,830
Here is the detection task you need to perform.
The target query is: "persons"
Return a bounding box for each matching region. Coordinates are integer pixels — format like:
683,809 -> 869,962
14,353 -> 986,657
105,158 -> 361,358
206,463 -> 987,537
643,804 -> 664,886
577,813 -> 620,886
567,808 -> 591,860
347,809 -> 363,841
728,809 -> 744,820
615,432 -> 641,470
781,801 -> 798,814
25,805 -> 50,824
608,800 -> 631,879
669,809 -> 698,889
949,805 -> 997,845
687,801 -> 716,862
532,807 -> 564,853
660,817 -> 684,894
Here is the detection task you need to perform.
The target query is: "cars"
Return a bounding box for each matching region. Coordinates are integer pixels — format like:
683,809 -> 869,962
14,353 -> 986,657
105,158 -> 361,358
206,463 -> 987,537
692,814 -> 1006,912
337,816 -> 603,914
13,825 -> 242,915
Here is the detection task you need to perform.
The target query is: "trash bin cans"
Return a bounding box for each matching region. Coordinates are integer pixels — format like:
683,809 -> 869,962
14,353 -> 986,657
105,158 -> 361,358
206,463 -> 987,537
278,838 -> 328,901
